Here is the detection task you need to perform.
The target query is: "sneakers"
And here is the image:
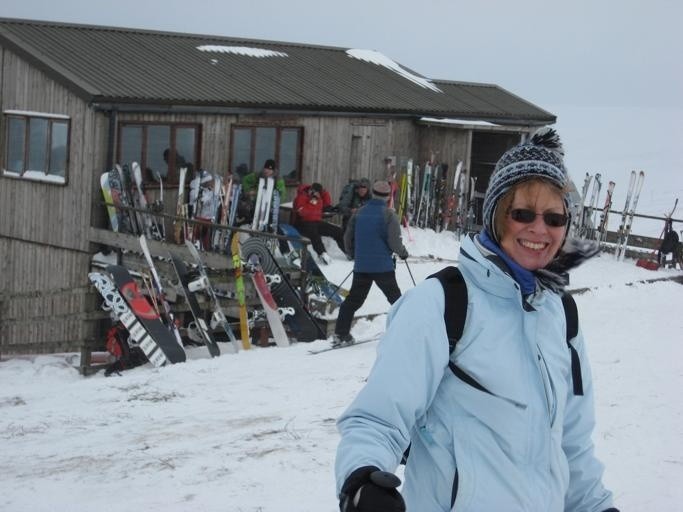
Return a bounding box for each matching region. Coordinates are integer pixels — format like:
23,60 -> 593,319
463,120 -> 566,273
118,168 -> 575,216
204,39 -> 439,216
319,252 -> 332,266
331,332 -> 355,347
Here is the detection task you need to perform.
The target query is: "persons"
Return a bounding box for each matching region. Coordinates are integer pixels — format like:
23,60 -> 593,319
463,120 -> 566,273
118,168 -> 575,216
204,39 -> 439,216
339,179 -> 375,232
332,180 -> 409,344
294,181 -> 348,269
239,157 -> 286,242
332,126 -> 620,512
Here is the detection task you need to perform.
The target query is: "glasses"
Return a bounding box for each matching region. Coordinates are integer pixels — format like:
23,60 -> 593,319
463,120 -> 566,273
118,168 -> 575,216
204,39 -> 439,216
504,205 -> 568,226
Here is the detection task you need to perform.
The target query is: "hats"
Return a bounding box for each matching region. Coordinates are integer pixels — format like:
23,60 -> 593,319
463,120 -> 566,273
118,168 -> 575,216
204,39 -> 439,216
263,159 -> 276,171
482,127 -> 571,259
373,181 -> 392,201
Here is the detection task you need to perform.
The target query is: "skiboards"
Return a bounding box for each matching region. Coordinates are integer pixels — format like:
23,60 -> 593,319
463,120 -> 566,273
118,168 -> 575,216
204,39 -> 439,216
186,232 -> 251,351
308,331 -> 383,355
138,235 -> 184,348
156,168 -> 274,254
574,170 -> 644,263
398,159 -> 477,240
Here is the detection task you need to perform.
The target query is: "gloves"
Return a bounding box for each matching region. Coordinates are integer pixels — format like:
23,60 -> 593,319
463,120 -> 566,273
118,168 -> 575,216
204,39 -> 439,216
334,468 -> 406,512
310,195 -> 320,206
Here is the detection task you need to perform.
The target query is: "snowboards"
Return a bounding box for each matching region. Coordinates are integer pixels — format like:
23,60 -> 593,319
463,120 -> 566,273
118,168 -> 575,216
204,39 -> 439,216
88,264 -> 185,368
279,224 -> 341,304
100,162 -> 152,239
168,250 -> 220,356
241,239 -> 327,348
